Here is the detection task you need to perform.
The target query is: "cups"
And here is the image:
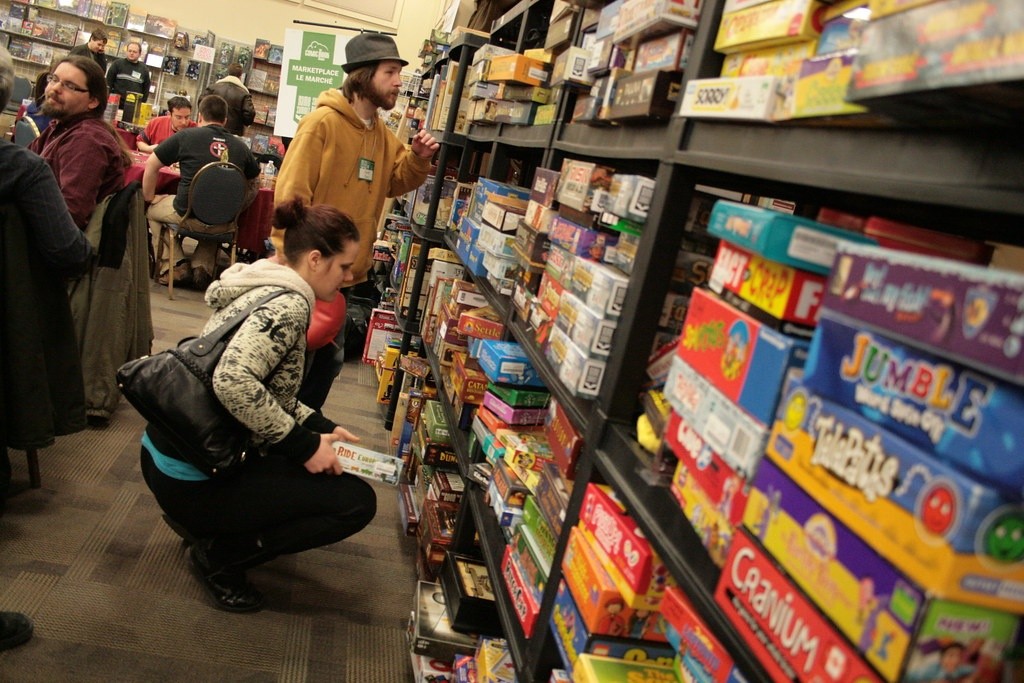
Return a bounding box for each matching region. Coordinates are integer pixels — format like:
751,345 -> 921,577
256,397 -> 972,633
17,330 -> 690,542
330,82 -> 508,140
115,110 -> 124,122
7,124 -> 16,132
3,131 -> 13,141
27,96 -> 35,102
20,99 -> 32,117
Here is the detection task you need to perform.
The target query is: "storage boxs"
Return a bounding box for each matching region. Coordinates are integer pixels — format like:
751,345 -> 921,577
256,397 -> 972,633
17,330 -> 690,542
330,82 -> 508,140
335,1 -> 1023,681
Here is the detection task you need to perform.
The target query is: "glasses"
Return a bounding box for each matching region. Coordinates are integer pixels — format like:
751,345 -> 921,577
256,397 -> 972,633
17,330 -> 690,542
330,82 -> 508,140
47,74 -> 90,92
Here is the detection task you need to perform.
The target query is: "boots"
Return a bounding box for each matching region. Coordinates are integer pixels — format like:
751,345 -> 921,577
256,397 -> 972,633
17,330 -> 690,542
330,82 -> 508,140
161,511 -> 202,544
191,535 -> 277,614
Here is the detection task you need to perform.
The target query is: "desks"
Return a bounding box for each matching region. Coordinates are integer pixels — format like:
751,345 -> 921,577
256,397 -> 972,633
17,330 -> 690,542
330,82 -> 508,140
113,128 -> 138,150
125,151 -> 275,264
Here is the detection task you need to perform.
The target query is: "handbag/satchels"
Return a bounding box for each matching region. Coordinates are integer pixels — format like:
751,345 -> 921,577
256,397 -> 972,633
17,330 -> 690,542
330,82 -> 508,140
115,290 -> 311,485
344,294 -> 378,363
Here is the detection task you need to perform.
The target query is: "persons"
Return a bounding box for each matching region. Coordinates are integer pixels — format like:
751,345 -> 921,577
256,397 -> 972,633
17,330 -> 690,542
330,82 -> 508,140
0,44 -> 90,448
142,95 -> 261,288
140,196 -> 377,612
272,33 -> 439,412
67,28 -> 108,74
137,97 -> 198,153
198,63 -> 256,137
25,55 -> 132,233
107,42 -> 151,123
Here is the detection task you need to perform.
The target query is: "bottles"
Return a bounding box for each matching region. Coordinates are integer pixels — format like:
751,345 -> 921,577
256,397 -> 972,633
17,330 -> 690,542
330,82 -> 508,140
263,160 -> 275,189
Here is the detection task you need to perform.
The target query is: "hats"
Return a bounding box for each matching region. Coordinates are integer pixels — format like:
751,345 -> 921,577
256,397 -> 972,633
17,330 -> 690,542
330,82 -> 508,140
341,33 -> 409,74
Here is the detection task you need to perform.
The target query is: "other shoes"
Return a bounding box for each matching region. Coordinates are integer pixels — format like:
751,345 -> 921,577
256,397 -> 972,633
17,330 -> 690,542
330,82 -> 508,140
0,611 -> 34,650
192,266 -> 213,290
159,259 -> 194,285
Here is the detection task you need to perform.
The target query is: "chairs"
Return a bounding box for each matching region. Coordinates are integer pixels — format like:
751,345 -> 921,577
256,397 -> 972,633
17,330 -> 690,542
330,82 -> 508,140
14,116 -> 41,149
152,161 -> 248,300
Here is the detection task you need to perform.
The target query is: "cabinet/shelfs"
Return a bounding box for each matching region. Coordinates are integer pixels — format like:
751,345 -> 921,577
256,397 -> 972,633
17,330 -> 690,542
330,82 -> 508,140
1,1 -> 288,157
413,1 -> 1023,682
379,32 -> 489,433
